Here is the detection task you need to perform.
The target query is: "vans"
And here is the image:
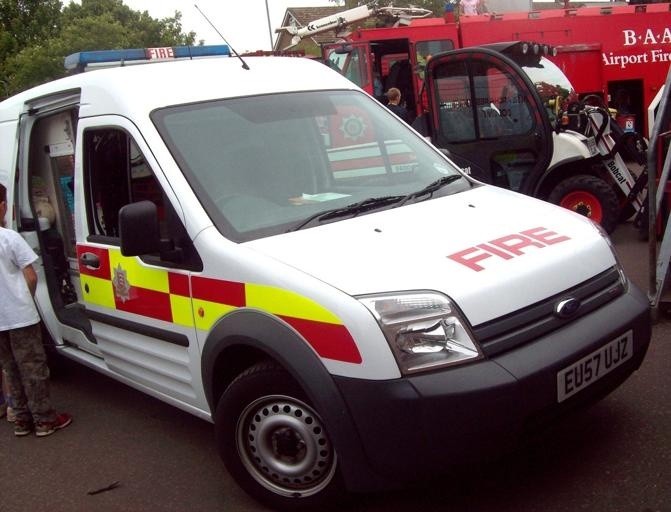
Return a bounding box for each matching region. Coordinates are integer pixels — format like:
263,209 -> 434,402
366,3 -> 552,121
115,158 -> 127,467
0,43 -> 654,511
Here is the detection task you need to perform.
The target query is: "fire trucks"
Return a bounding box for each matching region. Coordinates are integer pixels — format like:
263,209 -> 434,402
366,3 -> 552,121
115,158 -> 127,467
274,0 -> 671,240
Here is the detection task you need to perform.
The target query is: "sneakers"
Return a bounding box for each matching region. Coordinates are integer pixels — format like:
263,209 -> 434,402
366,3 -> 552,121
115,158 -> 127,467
35,411 -> 74,437
11,419 -> 33,436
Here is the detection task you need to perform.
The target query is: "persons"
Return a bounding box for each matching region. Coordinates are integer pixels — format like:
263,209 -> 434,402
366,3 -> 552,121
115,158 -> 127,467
459,1 -> 481,16
0,183 -> 73,438
385,87 -> 411,124
1,363 -> 19,423
